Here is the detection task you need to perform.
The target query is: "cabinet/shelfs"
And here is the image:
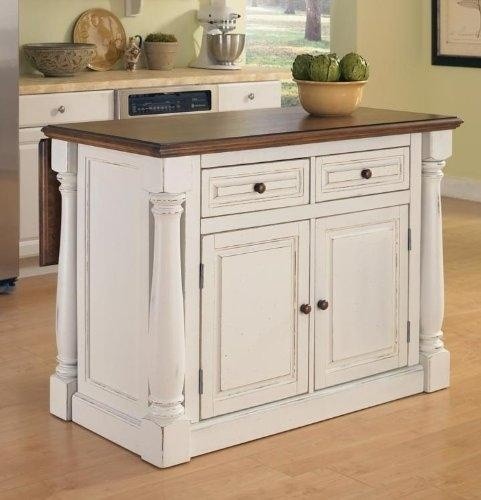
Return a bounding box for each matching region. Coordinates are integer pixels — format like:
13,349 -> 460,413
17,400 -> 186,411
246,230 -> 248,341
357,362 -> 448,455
18,89 -> 117,280
199,124 -> 413,457
219,80 -> 280,111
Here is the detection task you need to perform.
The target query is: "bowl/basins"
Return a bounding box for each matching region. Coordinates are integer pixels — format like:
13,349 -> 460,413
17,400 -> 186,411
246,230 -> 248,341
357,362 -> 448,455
293,78 -> 365,116
22,42 -> 96,76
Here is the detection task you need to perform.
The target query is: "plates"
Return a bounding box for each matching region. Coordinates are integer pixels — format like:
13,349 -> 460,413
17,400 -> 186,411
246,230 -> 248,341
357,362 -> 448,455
72,8 -> 127,72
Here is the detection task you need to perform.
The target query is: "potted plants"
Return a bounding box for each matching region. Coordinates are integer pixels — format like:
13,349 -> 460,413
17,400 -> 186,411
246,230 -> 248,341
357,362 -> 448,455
144,32 -> 177,69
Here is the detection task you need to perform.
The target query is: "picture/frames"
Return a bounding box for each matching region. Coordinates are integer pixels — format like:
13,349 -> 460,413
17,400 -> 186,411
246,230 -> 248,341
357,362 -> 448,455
431,0 -> 481,68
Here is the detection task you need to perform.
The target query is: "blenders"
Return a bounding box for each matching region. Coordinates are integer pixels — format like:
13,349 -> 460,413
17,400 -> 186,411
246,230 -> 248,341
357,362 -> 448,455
188,0 -> 245,70
